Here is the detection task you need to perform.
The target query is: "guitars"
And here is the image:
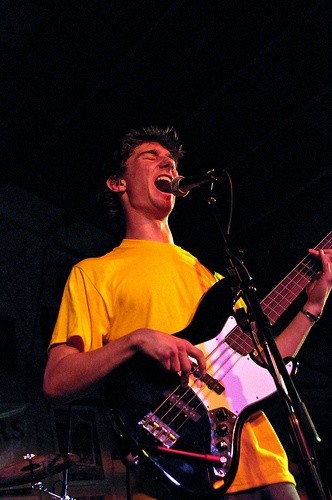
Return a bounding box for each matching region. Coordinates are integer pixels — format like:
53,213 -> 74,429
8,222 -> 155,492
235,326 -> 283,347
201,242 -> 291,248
97,222 -> 331,500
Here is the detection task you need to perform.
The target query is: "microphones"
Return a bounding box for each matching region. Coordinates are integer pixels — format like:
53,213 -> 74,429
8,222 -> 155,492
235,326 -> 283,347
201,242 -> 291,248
171,168 -> 226,197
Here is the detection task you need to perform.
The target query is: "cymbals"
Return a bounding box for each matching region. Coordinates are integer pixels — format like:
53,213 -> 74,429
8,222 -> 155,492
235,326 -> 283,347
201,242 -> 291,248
0,402 -> 31,420
0,452 -> 79,488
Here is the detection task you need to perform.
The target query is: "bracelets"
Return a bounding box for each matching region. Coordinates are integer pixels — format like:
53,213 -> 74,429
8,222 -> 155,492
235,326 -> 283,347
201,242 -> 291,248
300,308 -> 319,323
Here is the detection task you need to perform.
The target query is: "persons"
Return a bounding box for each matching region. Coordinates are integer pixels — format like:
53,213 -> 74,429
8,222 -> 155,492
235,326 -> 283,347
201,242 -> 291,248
41,127 -> 332,499
